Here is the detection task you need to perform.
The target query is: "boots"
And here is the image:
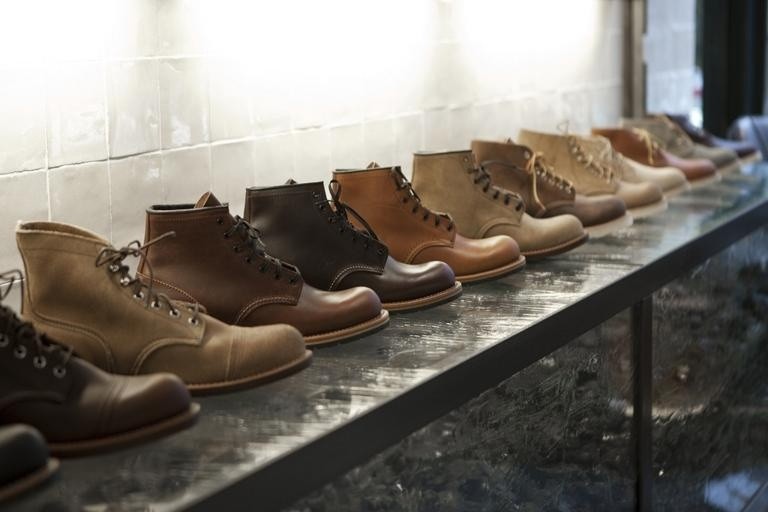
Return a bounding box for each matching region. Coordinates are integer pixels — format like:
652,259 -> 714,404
243,180 -> 462,311
134,191 -> 389,350
333,111 -> 762,283
16,221 -> 312,396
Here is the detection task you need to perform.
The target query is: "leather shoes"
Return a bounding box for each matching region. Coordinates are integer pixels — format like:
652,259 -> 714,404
0,269 -> 201,460
1,426 -> 59,501
